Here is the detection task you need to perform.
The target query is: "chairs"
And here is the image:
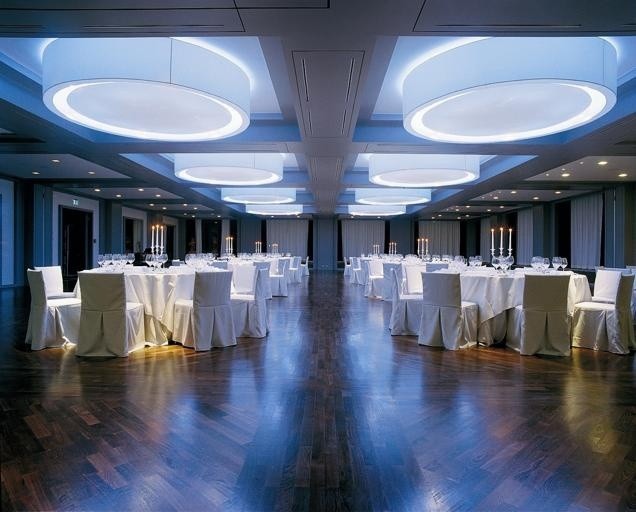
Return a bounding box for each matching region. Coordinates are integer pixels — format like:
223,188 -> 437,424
19,248 -> 313,358
338,252 -> 636,358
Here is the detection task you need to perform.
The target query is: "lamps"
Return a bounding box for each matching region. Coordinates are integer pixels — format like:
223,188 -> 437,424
219,186 -> 298,206
37,35 -> 252,143
391,35 -> 618,147
352,186 -> 433,207
171,150 -> 285,186
241,202 -> 305,216
346,203 -> 408,218
367,151 -> 480,188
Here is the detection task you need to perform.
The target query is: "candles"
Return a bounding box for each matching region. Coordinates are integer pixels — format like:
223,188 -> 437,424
253,240 -> 262,255
490,227 -> 513,256
271,244 -> 279,254
150,223 -> 164,256
373,244 -> 379,255
388,241 -> 396,255
415,238 -> 429,255
225,235 -> 234,255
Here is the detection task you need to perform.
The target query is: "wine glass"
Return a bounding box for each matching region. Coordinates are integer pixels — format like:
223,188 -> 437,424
359,253 -> 568,276
97,252 -> 135,272
183,251 -> 214,272
221,251 -> 283,263
146,252 -> 169,274
